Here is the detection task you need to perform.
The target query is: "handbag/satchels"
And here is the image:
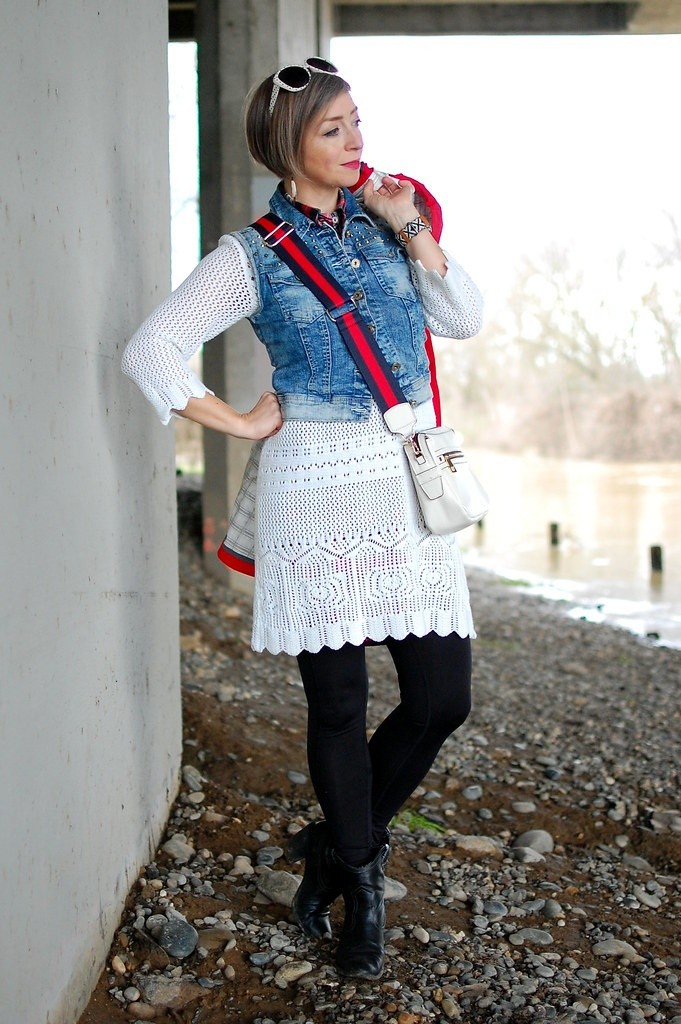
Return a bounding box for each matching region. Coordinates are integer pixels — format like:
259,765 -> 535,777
404,426 -> 489,536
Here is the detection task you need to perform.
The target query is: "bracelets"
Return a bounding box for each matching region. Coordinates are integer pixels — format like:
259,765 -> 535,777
396,215 -> 432,246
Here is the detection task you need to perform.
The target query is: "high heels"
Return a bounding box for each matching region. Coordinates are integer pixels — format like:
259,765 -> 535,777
287,819 -> 344,942
330,822 -> 391,980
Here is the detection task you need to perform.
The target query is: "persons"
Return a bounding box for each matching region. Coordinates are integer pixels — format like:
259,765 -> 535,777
121,65 -> 483,980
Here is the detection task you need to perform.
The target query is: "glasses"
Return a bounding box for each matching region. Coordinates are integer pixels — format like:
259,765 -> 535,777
269,56 -> 339,115
